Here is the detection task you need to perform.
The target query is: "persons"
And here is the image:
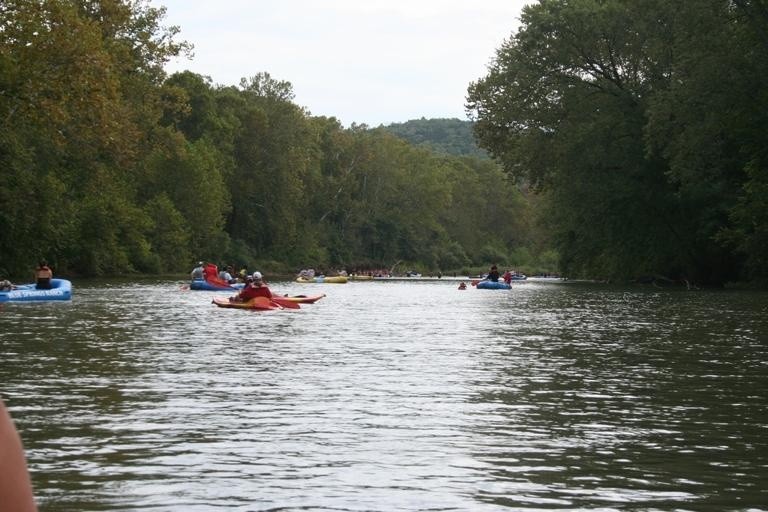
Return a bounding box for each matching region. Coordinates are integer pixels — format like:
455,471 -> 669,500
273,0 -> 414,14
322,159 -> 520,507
0,397 -> 39,512
34,257 -> 53,289
296,265 -> 457,280
486,265 -> 499,282
191,261 -> 272,302
503,270 -> 511,284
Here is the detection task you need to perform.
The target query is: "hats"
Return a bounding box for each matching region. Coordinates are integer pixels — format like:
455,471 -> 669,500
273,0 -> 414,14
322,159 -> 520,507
253,271 -> 264,280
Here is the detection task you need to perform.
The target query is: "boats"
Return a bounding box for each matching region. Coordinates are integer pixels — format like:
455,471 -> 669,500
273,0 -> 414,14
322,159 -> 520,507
296,275 -> 373,283
188,277 -> 326,310
469,274 -> 526,289
1,278 -> 74,301
